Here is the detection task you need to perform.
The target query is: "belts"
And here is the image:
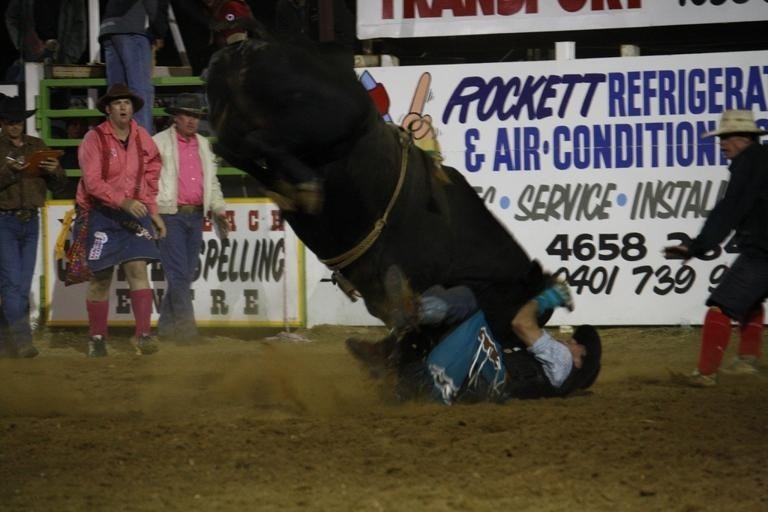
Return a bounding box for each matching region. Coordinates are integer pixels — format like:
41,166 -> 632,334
178,205 -> 203,214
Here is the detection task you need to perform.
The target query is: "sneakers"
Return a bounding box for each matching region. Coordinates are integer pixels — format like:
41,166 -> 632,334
135,331 -> 160,356
681,360 -> 756,387
17,343 -> 38,357
88,335 -> 109,357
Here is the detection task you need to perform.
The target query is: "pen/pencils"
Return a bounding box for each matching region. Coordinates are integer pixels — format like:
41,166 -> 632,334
6,156 -> 25,166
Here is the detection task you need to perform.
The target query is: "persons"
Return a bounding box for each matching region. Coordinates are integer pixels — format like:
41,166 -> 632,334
192,0 -> 255,76
0,95 -> 68,357
97,0 -> 169,136
338,266 -> 603,404
151,93 -> 232,347
661,109 -> 768,387
75,83 -> 168,358
5,0 -> 89,84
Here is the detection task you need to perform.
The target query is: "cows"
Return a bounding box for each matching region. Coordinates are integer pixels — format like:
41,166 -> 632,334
163,0 -> 573,407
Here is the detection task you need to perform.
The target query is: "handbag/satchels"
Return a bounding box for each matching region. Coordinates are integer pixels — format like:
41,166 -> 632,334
54,211 -> 90,286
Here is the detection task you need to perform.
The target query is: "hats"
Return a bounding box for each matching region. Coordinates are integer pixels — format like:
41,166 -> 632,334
96,82 -> 145,115
700,109 -> 768,139
165,92 -> 208,115
554,326 -> 601,397
0,96 -> 38,127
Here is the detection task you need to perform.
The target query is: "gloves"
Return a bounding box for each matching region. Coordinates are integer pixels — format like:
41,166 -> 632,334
535,283 -> 574,313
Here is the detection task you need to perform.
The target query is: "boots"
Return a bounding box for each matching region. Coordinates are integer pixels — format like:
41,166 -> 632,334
384,265 -> 447,323
